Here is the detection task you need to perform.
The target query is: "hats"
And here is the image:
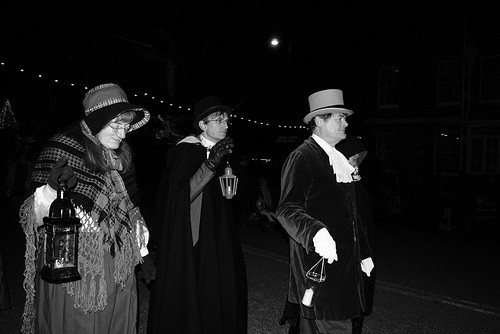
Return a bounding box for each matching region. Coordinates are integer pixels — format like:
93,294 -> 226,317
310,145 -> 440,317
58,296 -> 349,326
303,89 -> 354,125
82,83 -> 151,136
192,96 -> 232,132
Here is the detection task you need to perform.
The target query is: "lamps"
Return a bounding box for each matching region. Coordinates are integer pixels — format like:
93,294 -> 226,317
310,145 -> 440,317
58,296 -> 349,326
42,174 -> 84,284
217,157 -> 240,202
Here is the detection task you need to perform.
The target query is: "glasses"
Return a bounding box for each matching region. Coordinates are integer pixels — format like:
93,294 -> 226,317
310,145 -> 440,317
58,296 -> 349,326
203,115 -> 230,128
106,122 -> 135,134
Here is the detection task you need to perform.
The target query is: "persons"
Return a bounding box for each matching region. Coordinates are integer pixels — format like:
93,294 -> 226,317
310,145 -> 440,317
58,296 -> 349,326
275,88 -> 377,334
19,83 -> 155,334
336,135 -> 369,166
146,98 -> 249,334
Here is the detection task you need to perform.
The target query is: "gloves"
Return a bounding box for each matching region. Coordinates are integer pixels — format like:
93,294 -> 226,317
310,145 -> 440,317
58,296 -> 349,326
47,156 -> 77,193
205,136 -> 234,172
360,256 -> 375,277
313,225 -> 338,264
